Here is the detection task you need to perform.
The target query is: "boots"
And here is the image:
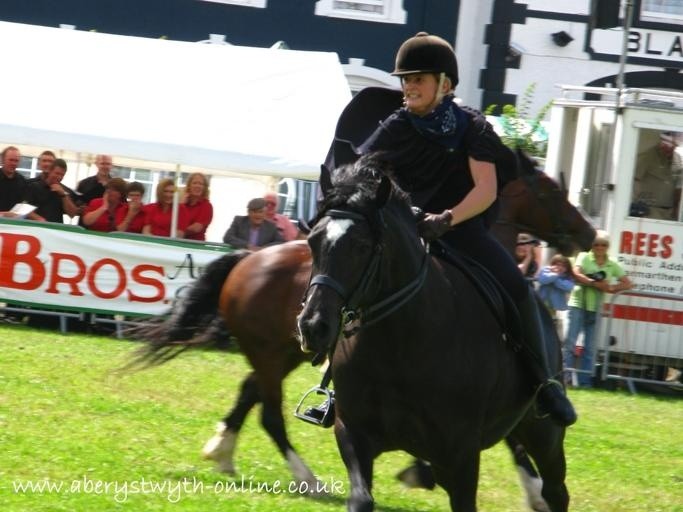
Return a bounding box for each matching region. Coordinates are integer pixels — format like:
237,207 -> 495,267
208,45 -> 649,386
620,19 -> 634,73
516,284 -> 576,424
304,396 -> 335,419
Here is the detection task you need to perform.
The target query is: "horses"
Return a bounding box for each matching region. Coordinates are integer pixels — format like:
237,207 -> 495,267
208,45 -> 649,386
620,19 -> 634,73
108,144 -> 599,502
294,149 -> 574,512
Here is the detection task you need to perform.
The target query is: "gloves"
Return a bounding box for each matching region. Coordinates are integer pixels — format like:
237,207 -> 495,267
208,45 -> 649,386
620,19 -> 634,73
415,210 -> 455,242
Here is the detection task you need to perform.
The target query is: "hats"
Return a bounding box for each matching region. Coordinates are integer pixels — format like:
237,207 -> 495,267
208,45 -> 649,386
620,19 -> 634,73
389,32 -> 458,89
515,232 -> 540,245
247,198 -> 265,211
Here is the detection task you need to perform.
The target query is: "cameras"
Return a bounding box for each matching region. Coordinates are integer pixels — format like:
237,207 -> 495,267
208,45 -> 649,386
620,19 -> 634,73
586,270 -> 606,281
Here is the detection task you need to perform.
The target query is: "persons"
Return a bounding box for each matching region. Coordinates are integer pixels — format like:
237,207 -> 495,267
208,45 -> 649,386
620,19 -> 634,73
222,198 -> 285,251
0,145 -> 214,243
260,194 -> 299,241
304,30 -> 577,430
563,232 -> 630,383
634,133 -> 683,220
515,232 -> 542,281
535,251 -> 574,336
